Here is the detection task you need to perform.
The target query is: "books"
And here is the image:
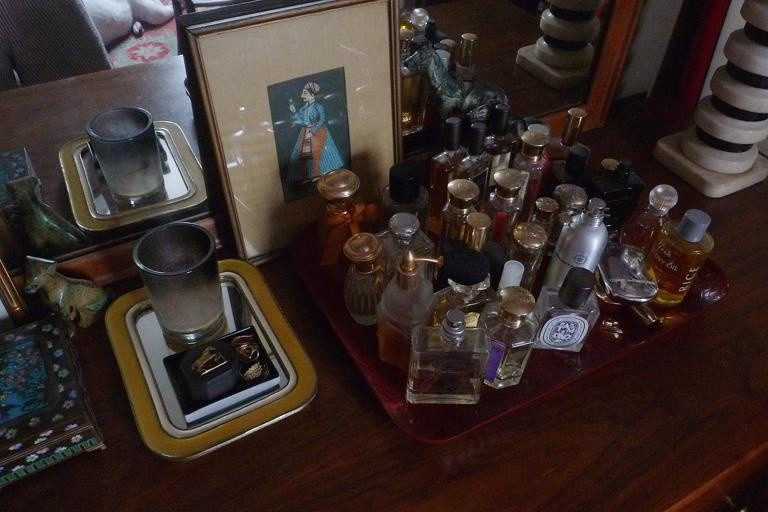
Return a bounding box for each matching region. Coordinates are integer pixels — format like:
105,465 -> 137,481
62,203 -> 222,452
162,323 -> 283,429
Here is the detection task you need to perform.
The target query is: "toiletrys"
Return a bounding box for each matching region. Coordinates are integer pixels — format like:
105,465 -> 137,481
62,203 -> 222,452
397,8 -> 509,140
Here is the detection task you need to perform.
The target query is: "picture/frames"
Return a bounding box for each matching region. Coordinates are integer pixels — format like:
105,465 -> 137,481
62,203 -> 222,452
181,1 -> 402,265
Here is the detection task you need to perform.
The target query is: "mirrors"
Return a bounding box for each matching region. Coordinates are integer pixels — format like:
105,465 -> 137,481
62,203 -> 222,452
0,0 -> 643,307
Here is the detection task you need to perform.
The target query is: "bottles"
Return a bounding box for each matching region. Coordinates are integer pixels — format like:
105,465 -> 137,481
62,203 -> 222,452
397,0 -> 482,136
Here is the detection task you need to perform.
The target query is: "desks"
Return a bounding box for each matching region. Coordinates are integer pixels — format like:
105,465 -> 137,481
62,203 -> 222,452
0,2 -> 588,251
1,154 -> 766,511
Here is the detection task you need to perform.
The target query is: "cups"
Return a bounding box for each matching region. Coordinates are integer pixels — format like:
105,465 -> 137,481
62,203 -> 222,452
133,220 -> 226,352
85,103 -> 166,211
